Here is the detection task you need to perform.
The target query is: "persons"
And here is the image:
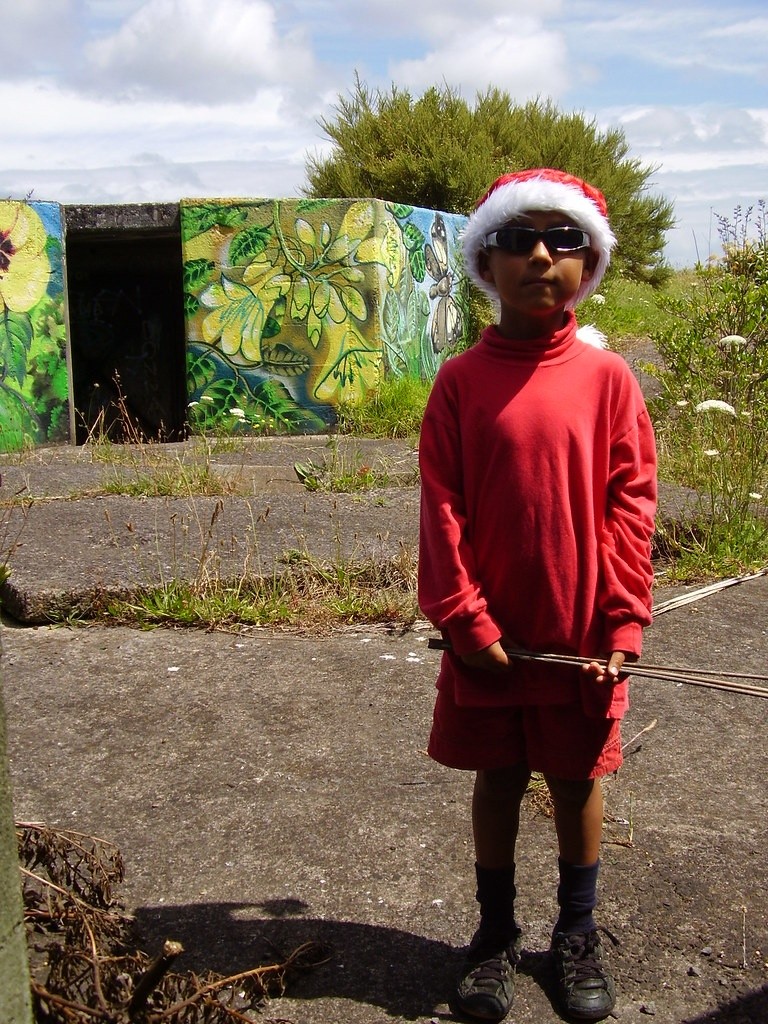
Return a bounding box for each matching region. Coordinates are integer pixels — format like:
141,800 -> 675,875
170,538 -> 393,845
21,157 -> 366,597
417,169 -> 659,1021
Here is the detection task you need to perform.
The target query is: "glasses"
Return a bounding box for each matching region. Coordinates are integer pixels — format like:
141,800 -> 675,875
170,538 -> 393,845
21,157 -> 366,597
483,226 -> 596,253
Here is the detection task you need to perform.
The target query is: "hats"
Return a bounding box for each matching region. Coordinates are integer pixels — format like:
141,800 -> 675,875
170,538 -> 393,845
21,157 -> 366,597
455,169 -> 616,313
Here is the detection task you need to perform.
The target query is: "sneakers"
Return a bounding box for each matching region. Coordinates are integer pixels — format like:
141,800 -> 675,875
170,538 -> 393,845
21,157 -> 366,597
546,923 -> 620,1020
455,923 -> 523,1024
